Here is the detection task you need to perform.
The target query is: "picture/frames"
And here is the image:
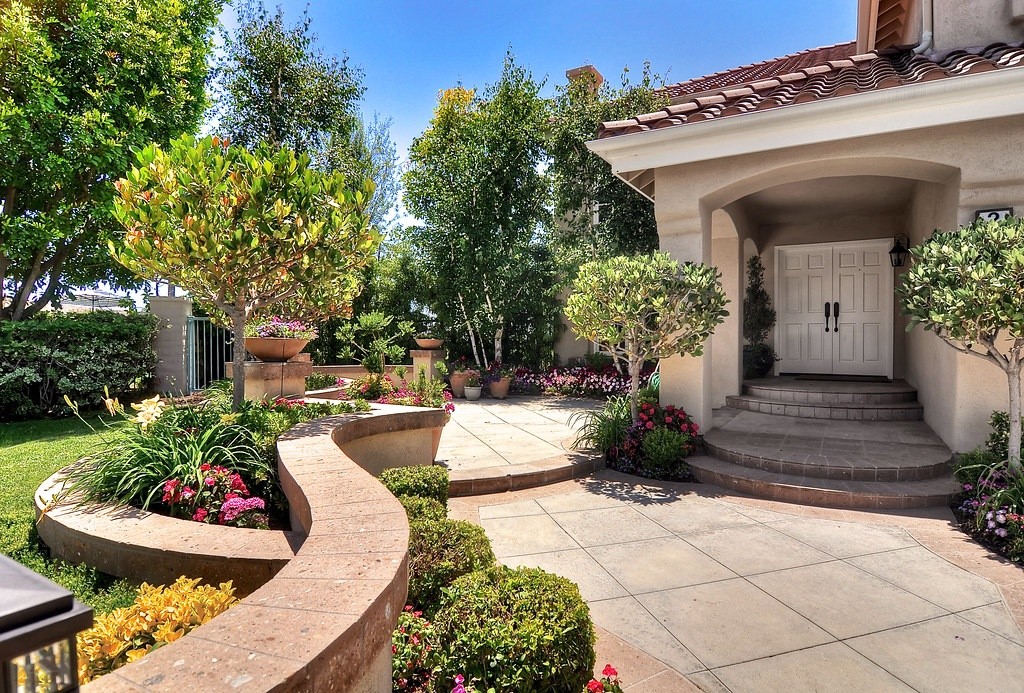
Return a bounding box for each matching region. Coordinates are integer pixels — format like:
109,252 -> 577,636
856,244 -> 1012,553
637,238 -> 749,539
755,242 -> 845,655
975,207 -> 1014,222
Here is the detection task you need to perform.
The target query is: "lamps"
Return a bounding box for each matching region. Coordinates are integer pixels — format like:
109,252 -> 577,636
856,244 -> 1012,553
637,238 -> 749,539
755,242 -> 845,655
888,233 -> 910,268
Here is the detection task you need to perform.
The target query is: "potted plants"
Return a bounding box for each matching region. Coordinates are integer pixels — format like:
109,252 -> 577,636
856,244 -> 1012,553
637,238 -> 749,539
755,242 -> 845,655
743,256 -> 782,378
489,360 -> 528,399
413,330 -> 444,350
445,356 -> 486,397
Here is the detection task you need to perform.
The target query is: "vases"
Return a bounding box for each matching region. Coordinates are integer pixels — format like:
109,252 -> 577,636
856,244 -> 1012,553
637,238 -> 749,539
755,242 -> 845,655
244,337 -> 308,362
464,387 -> 482,401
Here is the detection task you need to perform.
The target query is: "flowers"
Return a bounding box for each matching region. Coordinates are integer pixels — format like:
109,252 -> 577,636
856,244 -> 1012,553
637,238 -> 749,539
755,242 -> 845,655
464,369 -> 487,386
226,317 -> 319,344
